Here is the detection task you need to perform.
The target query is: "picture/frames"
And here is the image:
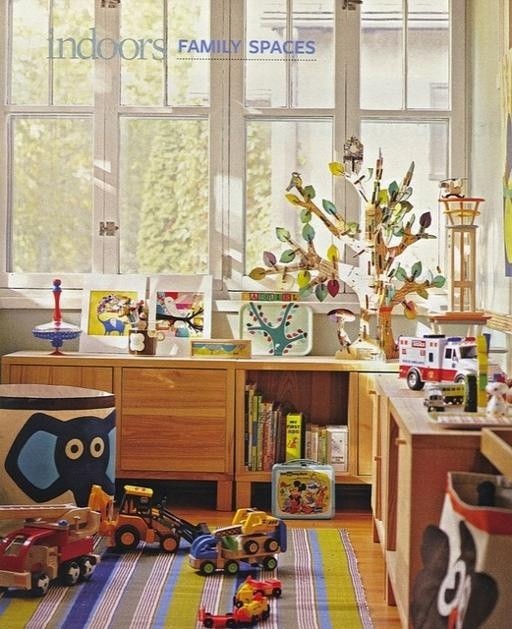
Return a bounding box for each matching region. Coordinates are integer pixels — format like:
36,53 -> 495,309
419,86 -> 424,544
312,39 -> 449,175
148,273 -> 212,341
78,272 -> 147,354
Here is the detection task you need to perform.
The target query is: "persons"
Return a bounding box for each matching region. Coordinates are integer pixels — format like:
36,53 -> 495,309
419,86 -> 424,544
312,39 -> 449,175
0,479 -> 291,628
1,480 -> 291,629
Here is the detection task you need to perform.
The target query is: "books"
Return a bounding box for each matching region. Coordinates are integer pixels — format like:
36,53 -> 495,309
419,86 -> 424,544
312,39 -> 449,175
241,380 -> 346,471
